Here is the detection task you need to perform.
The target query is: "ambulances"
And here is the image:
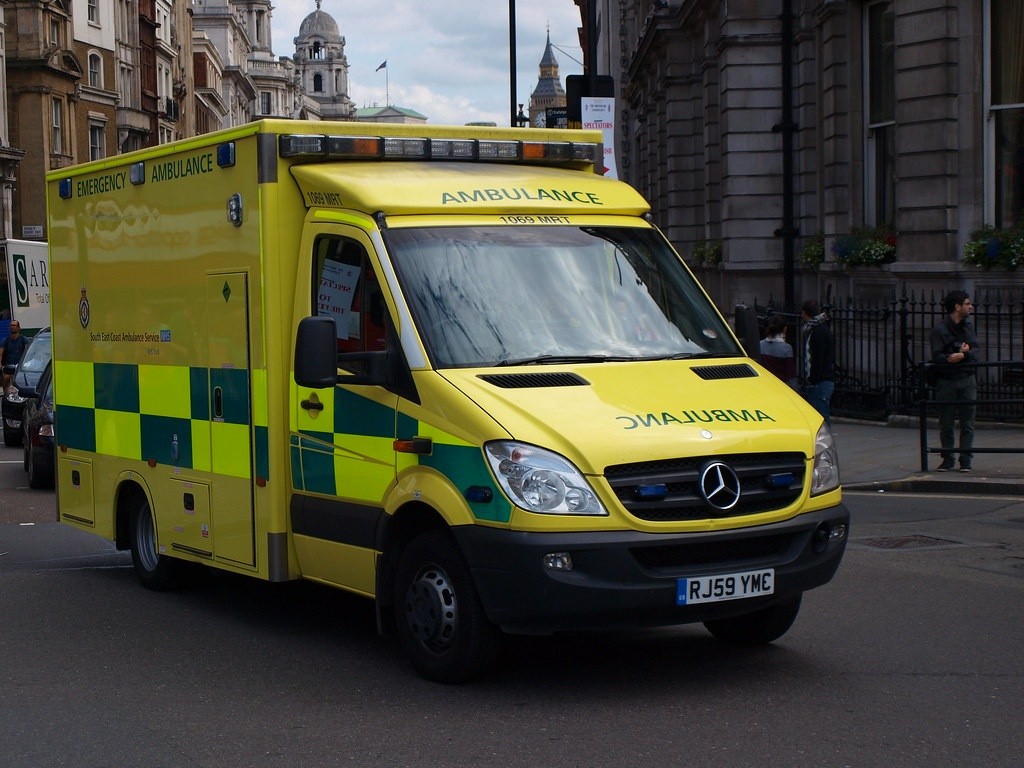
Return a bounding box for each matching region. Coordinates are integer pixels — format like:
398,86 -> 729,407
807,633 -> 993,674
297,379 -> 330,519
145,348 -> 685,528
45,119 -> 850,687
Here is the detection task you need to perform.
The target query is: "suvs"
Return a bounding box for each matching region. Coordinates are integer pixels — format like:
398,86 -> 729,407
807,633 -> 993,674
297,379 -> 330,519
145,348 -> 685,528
2,326 -> 52,447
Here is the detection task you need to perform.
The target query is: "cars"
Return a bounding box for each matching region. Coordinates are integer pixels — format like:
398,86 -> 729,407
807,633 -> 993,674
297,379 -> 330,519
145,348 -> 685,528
18,358 -> 55,492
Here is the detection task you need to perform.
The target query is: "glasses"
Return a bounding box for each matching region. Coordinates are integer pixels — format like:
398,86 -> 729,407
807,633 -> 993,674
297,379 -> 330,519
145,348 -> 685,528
960,302 -> 971,306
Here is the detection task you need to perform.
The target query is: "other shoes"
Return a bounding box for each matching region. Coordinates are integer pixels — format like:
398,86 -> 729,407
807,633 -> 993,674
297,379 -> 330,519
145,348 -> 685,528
938,462 -> 954,471
960,466 -> 972,472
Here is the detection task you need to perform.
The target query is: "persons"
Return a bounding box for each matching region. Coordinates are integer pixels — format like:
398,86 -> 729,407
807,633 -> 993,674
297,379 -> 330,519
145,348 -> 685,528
760,314 -> 799,390
930,290 -> 980,472
0,320 -> 30,394
801,300 -> 837,432
